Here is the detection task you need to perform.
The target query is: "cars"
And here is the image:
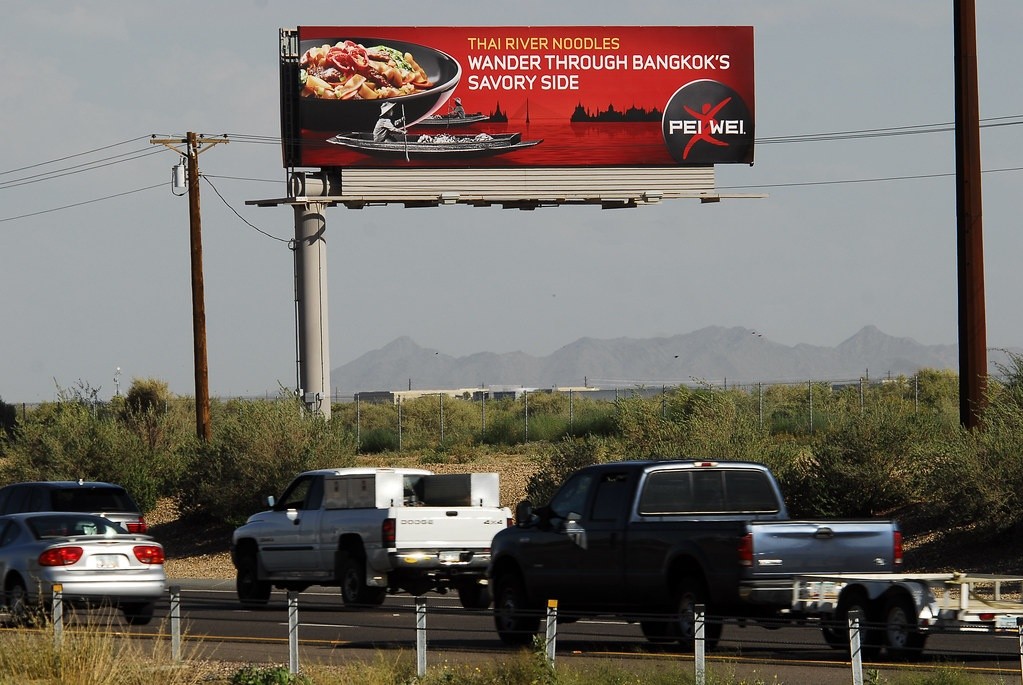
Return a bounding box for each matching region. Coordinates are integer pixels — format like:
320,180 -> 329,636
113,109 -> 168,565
0,511 -> 166,627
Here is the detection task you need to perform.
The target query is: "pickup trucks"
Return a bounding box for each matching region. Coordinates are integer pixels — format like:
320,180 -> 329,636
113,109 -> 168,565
229,468 -> 516,614
488,454 -> 903,654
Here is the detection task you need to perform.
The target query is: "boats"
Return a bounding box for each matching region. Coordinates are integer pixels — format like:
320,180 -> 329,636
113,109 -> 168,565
416,111 -> 492,127
323,129 -> 545,163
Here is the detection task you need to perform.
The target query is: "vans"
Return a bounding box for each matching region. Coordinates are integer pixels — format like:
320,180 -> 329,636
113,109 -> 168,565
1,479 -> 148,539
